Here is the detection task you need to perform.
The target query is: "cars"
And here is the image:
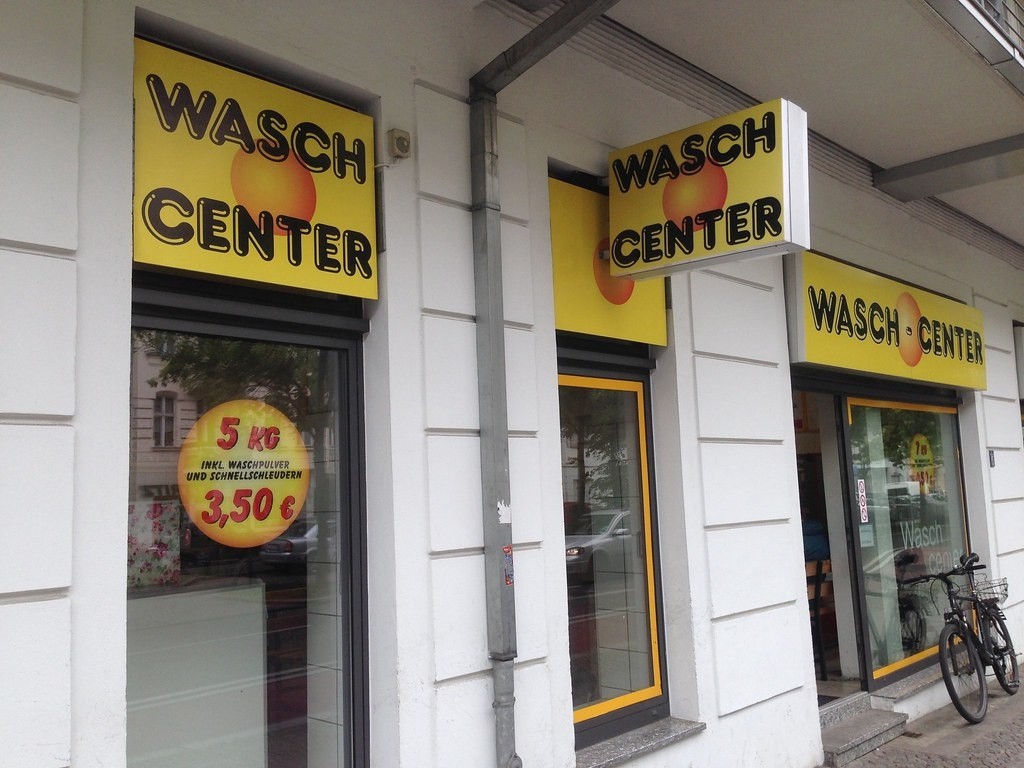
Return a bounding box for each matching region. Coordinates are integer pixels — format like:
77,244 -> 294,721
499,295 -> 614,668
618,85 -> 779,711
564,507 -> 641,587
260,517 -> 340,573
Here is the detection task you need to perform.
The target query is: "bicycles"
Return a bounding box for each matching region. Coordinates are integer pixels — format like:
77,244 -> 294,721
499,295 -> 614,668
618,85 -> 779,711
863,549 -> 931,669
903,552 -> 1021,725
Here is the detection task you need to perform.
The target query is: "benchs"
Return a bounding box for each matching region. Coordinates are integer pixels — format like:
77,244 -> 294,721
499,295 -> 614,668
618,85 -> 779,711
806,560 -> 836,681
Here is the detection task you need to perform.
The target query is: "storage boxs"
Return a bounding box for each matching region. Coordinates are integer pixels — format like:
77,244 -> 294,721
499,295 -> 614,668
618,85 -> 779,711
127,499 -> 182,591
